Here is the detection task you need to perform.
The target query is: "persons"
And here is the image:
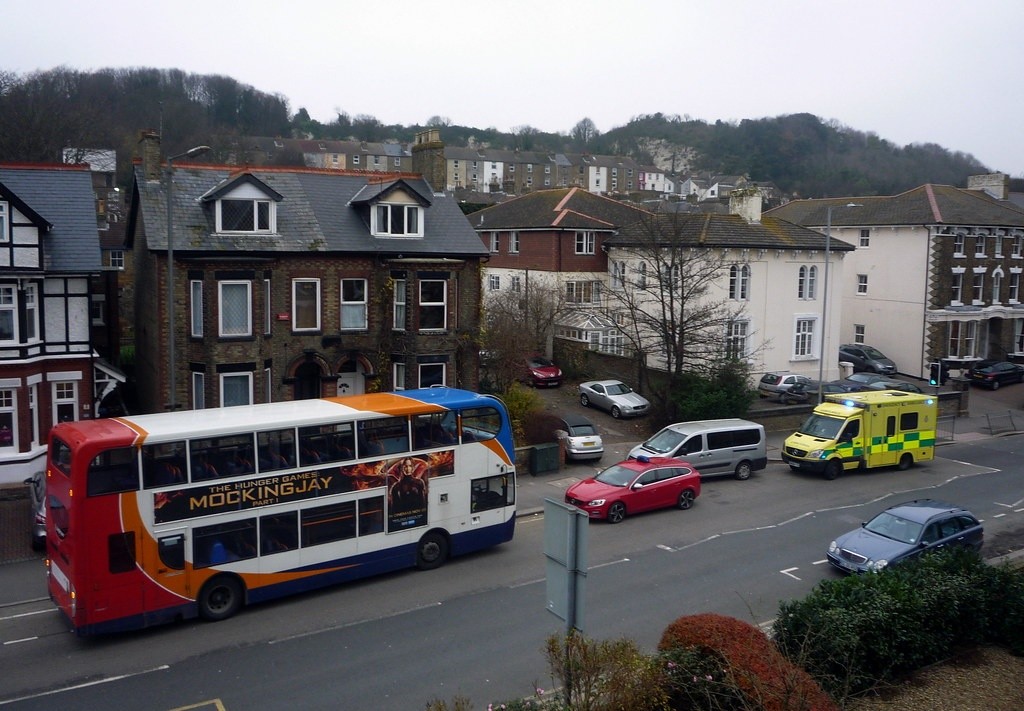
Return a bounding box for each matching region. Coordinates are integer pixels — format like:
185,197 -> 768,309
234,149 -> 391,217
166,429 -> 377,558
389,457 -> 426,514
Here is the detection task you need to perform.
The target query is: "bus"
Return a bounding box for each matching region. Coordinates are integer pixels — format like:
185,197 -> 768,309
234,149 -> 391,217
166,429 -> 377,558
46,385 -> 518,640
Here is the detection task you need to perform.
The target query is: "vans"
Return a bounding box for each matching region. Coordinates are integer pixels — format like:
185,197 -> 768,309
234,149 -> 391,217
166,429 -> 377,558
627,418 -> 768,481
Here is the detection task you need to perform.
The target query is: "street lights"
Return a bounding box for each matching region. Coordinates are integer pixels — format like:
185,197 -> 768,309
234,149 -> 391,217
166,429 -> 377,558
819,203 -> 863,402
164,146 -> 213,410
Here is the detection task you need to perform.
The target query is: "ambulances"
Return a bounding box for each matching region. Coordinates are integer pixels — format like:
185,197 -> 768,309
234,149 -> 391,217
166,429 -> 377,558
780,389 -> 938,480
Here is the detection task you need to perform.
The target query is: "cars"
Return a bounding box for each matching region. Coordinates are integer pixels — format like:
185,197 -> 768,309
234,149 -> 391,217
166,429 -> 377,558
565,456 -> 702,524
542,414 -> 605,463
967,358 -> 1024,389
825,497 -> 984,577
509,351 -> 566,388
578,380 -> 651,418
838,343 -> 897,375
757,371 -> 924,403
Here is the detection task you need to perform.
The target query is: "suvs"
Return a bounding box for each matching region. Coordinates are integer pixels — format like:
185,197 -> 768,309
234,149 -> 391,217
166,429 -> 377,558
24,471 -> 50,552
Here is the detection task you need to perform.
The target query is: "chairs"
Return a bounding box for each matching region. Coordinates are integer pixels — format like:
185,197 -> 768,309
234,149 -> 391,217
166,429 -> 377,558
159,443 -> 383,485
